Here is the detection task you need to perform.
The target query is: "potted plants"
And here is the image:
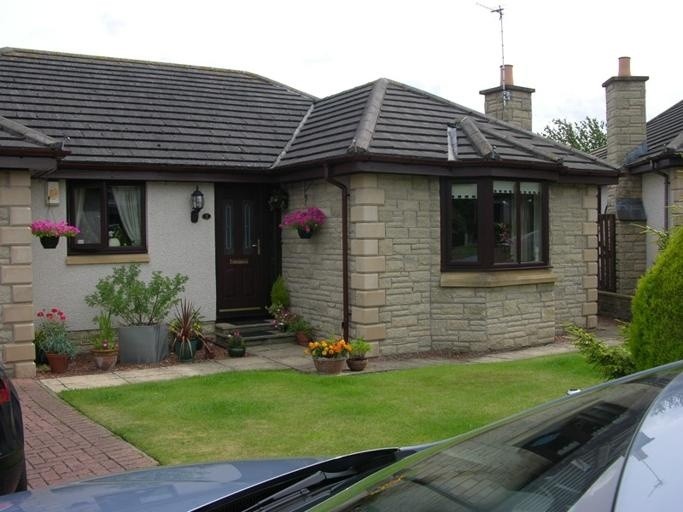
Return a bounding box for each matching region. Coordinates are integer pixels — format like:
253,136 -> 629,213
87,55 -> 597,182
86,311 -> 119,370
167,297 -> 210,362
346,337 -> 371,371
288,319 -> 317,346
84,263 -> 190,363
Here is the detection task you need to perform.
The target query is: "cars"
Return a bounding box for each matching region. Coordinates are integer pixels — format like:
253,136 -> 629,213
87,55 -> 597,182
0,359 -> 683,512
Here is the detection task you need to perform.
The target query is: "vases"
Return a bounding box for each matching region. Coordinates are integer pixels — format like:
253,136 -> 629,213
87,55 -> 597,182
47,353 -> 68,374
297,226 -> 313,239
39,236 -> 59,248
229,347 -> 245,358
312,354 -> 349,375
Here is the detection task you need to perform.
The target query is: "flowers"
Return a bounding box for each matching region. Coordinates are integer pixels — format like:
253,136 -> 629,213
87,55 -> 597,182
280,207 -> 327,233
228,329 -> 246,348
32,218 -> 80,239
303,335 -> 352,360
36,308 -> 73,356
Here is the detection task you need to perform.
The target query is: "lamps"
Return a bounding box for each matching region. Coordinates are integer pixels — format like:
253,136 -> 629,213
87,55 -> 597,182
190,185 -> 203,223
